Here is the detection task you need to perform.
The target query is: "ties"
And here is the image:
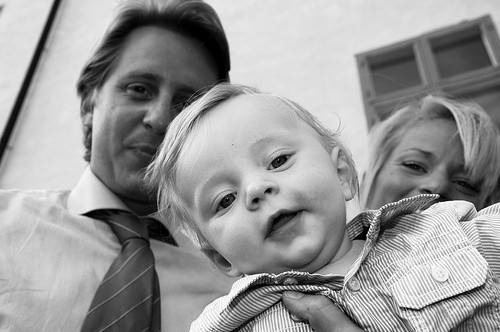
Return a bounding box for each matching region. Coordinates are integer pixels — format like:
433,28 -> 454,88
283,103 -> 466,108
80,208 -> 178,332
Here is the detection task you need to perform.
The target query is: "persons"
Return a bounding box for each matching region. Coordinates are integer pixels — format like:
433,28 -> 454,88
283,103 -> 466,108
355,92 -> 500,213
143,82 -> 500,332
0,0 -> 244,332
